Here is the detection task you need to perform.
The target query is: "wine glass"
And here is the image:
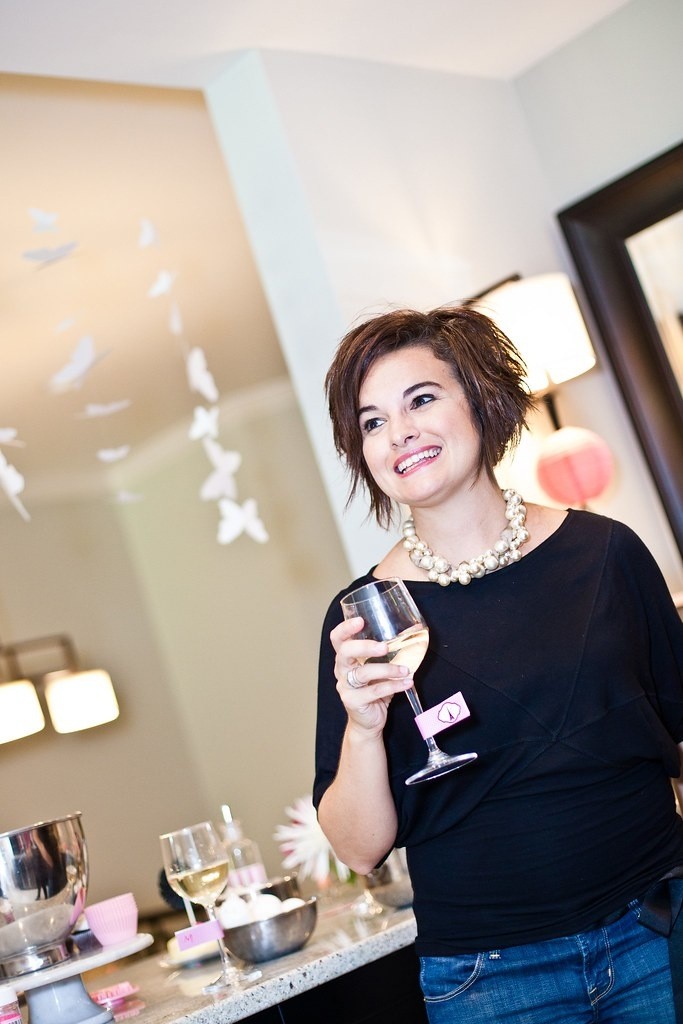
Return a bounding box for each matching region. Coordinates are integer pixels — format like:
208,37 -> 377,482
341,576 -> 477,786
158,820 -> 261,993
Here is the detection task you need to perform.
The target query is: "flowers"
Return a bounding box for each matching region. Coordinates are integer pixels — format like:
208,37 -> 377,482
272,799 -> 357,892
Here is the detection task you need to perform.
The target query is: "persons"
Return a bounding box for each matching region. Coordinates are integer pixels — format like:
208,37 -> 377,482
311,310 -> 683,1024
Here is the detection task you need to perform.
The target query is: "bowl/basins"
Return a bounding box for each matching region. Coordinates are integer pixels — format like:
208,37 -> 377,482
222,897 -> 317,964
1,812 -> 89,979
365,846 -> 415,909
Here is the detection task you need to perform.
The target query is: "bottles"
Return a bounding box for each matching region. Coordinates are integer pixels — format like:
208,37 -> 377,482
218,821 -> 268,889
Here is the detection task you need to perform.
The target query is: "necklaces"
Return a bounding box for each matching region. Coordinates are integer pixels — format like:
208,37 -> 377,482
403,488 -> 528,586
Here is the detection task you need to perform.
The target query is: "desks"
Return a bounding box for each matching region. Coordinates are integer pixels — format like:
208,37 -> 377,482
81,886 -> 418,1024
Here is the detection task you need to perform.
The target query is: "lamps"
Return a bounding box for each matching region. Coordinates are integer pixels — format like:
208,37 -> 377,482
0,633 -> 121,746
457,272 -> 615,515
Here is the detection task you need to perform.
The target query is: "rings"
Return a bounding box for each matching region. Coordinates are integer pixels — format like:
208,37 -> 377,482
347,668 -> 369,688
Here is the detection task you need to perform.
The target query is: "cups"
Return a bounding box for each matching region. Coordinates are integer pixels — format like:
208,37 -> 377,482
84,893 -> 139,947
